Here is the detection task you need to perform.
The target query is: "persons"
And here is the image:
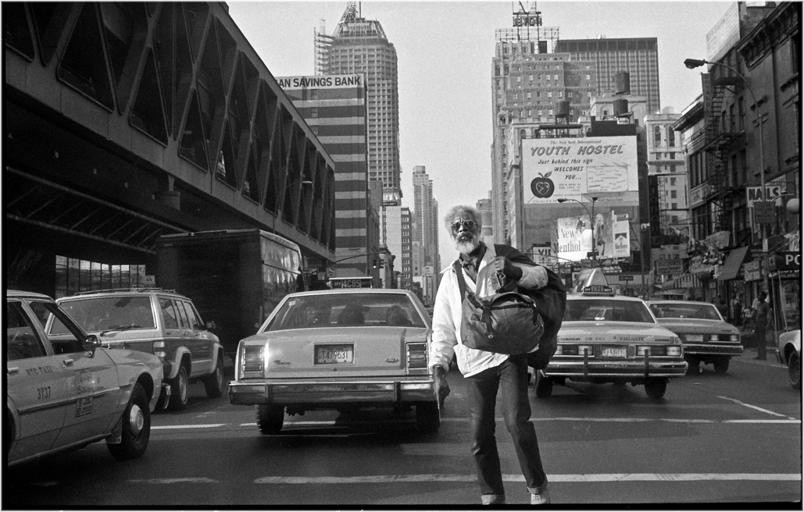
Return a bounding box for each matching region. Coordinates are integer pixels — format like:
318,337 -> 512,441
685,286 -> 744,326
428,204 -> 548,506
750,290 -> 770,360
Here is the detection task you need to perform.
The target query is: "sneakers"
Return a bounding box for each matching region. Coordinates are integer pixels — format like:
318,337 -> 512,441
529,488 -> 551,504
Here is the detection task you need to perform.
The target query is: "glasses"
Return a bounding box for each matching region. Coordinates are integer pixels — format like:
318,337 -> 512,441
452,220 -> 475,230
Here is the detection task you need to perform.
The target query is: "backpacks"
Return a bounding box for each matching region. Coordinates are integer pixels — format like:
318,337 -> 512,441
495,246 -> 567,370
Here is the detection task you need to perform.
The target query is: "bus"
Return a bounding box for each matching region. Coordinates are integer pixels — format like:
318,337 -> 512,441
153,229 -> 307,371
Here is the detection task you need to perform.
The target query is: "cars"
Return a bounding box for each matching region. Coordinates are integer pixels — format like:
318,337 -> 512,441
232,273 -> 450,440
5,280 -> 168,477
526,264 -> 801,405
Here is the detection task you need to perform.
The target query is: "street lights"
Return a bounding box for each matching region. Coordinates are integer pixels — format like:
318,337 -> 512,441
309,250 -> 387,274
554,194 -> 602,266
271,179 -> 314,240
677,57 -> 773,350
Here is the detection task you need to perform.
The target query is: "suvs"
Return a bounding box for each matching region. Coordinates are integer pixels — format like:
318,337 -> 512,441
45,284 -> 226,408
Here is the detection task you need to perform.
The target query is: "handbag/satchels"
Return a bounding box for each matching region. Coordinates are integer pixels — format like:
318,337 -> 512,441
461,291 -> 545,355
